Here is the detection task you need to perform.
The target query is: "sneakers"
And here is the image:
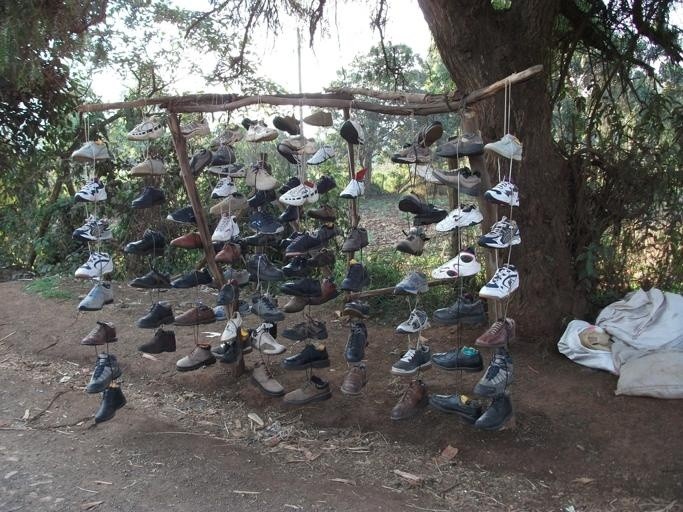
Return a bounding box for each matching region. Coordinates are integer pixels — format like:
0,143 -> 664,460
410,164 -> 444,184
477,216 -> 521,248
484,134 -> 521,162
252,363 -> 285,396
436,130 -> 483,158
414,121 -> 443,145
72,141 -> 111,162
431,249 -> 481,279
86,352 -> 121,393
95,387 -> 127,423
434,205 -> 483,232
283,376 -> 331,405
479,263 -> 519,301
398,191 -> 427,214
75,252 -> 114,279
395,309 -> 431,333
74,178 -> 107,203
397,232 -> 424,256
395,270 -> 430,295
80,321 -> 118,346
391,141 -> 430,163
78,282 -> 113,311
392,346 -> 430,375
473,355 -> 515,397
341,366 -> 367,396
483,180 -> 520,208
432,166 -> 481,196
412,204 -> 447,226
72,215 -> 113,242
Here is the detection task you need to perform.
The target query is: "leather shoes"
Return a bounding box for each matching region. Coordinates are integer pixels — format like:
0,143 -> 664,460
126,110 -> 371,372
431,343 -> 481,371
391,381 -> 426,420
428,392 -> 480,422
432,294 -> 485,325
476,398 -> 513,429
474,318 -> 516,349
